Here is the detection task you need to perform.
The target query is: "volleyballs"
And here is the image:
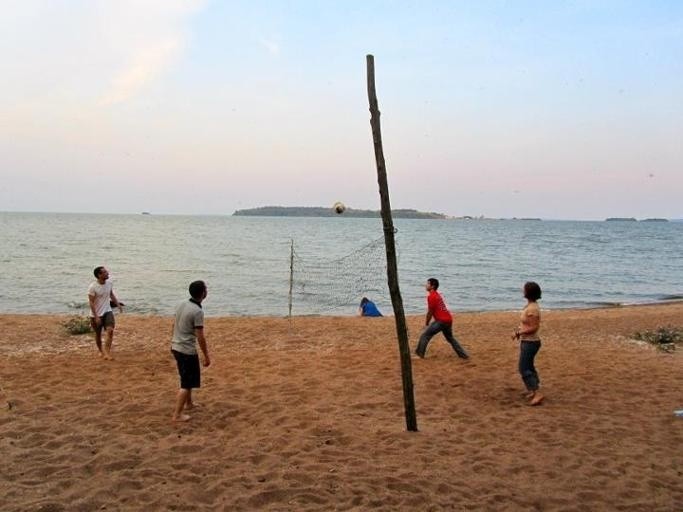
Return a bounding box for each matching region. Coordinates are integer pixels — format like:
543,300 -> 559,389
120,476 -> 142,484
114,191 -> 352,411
333,202 -> 344,214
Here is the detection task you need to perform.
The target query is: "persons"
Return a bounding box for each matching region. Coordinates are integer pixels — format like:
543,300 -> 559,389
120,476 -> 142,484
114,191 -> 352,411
87,267 -> 124,361
410,278 -> 468,360
511,282 -> 543,405
169,280 -> 210,422
357,296 -> 382,317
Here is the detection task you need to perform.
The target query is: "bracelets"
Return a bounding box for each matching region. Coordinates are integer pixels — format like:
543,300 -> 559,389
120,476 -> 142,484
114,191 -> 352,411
515,330 -> 519,340
